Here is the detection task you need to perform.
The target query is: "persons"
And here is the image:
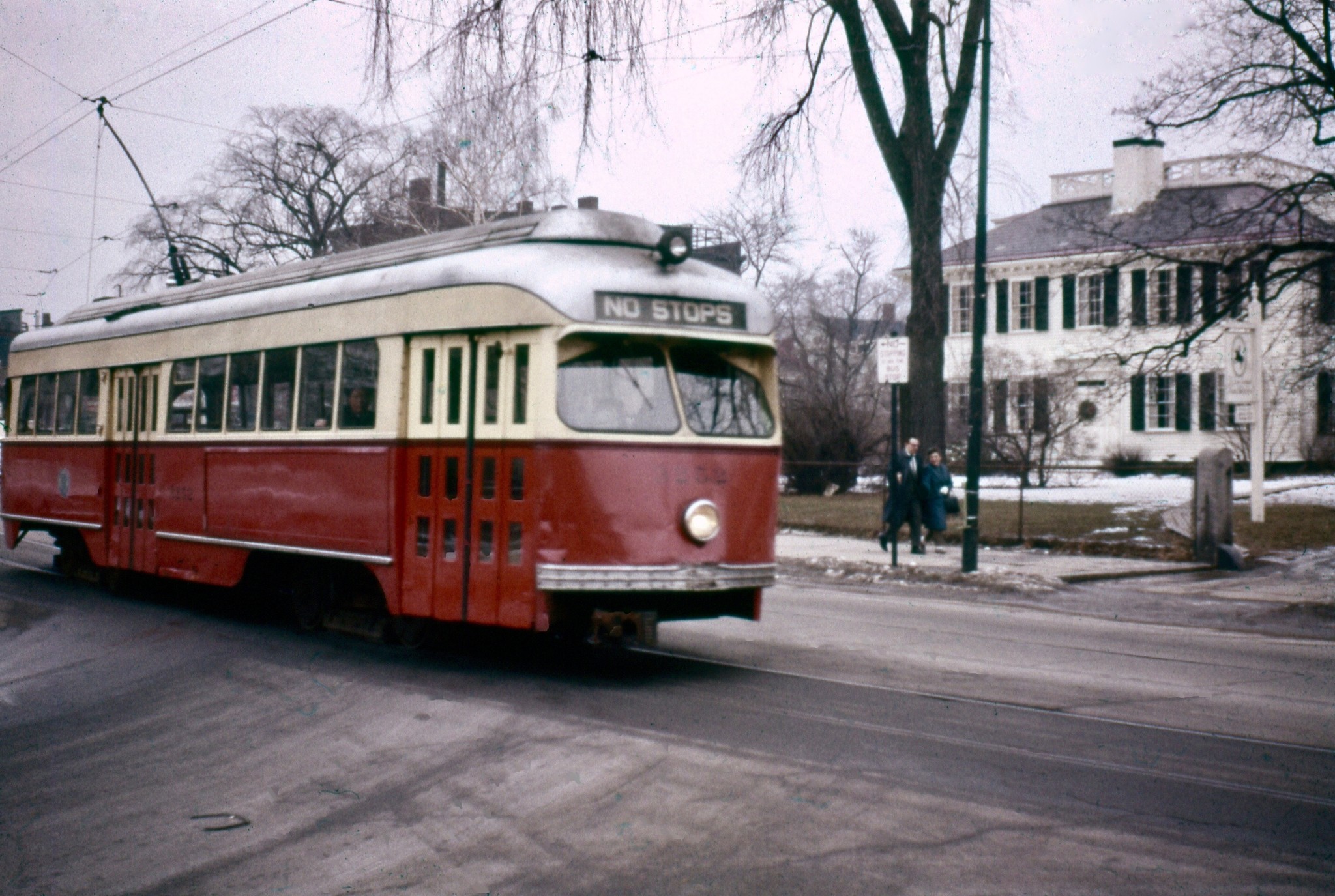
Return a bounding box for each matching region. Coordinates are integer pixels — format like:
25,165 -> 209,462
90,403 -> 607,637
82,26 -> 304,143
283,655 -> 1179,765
284,378 -> 376,430
878,437 -> 953,554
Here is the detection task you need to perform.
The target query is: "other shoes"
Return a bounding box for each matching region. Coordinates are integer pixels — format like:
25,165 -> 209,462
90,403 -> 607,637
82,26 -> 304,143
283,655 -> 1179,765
878,532 -> 888,551
911,546 -> 924,553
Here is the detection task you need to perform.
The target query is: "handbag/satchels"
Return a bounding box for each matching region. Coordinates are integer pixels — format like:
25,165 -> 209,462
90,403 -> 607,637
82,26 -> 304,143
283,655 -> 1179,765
943,493 -> 961,514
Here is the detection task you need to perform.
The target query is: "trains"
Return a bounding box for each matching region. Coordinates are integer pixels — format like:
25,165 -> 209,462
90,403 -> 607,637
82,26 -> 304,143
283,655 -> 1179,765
2,206 -> 782,652
